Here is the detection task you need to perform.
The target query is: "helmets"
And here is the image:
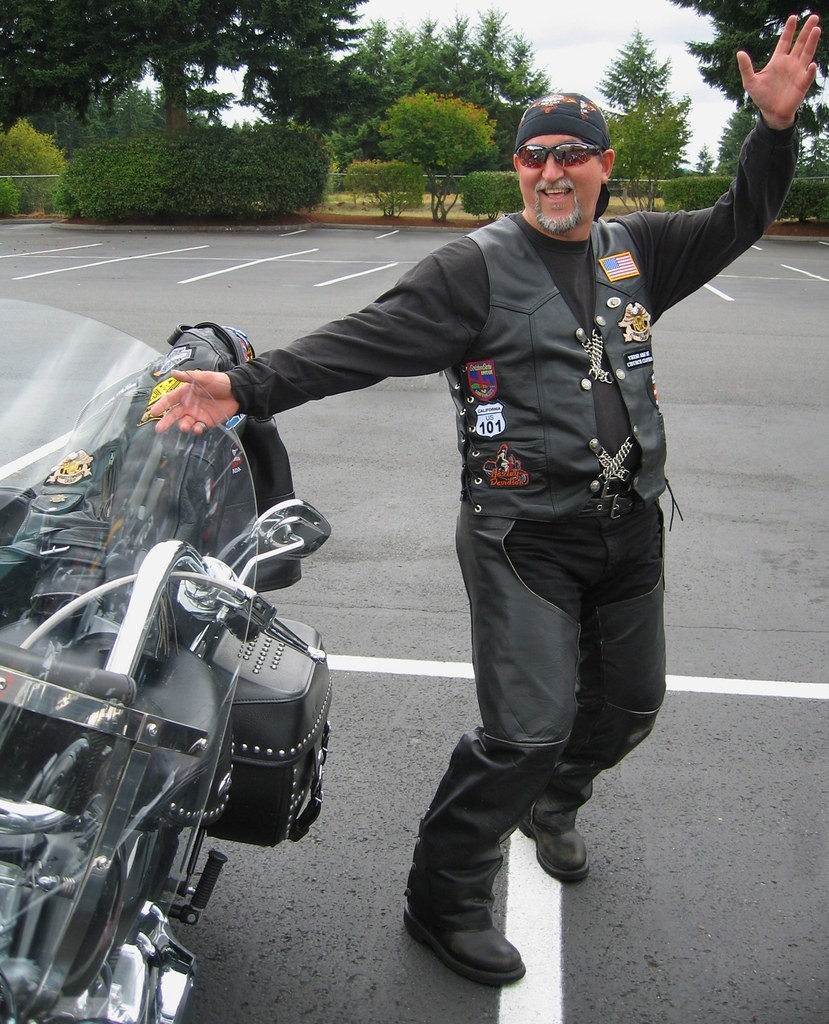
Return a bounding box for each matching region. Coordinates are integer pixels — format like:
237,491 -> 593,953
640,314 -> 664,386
193,319 -> 253,367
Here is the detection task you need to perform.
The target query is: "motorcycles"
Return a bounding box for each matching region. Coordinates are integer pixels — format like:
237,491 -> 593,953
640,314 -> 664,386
0,298 -> 332,1024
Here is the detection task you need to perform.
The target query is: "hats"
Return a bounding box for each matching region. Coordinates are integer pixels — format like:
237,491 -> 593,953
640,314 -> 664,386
514,93 -> 611,219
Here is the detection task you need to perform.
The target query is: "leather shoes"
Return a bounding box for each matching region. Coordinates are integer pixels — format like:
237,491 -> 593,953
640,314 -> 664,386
517,816 -> 591,881
403,903 -> 526,986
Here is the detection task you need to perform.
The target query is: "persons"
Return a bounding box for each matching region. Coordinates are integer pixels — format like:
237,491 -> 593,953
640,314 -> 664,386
151,14 -> 821,986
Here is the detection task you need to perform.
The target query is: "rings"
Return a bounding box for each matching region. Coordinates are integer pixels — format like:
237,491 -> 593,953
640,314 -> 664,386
196,421 -> 208,432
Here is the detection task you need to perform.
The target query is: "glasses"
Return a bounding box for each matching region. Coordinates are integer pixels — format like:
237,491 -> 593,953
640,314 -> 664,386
515,142 -> 600,168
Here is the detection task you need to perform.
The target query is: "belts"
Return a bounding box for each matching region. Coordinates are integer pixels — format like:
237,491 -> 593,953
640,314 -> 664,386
577,493 -> 646,519
